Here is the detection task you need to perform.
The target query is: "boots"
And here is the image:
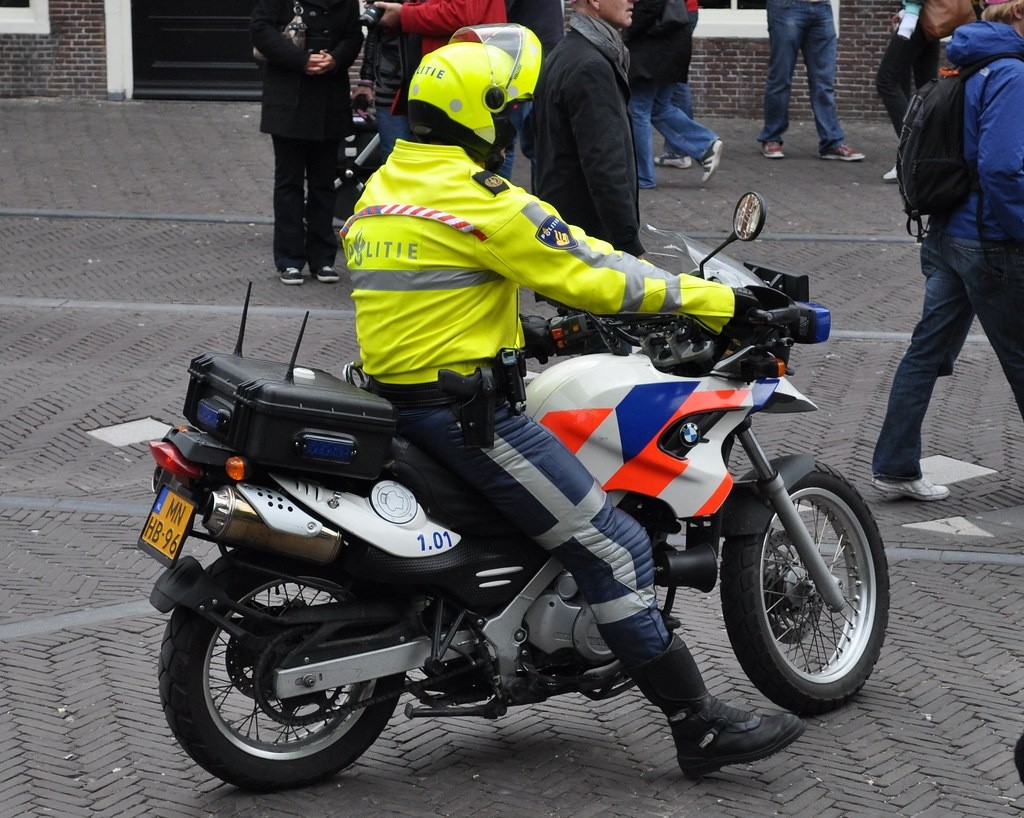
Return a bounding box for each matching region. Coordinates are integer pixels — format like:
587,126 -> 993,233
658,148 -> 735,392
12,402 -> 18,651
621,634 -> 803,778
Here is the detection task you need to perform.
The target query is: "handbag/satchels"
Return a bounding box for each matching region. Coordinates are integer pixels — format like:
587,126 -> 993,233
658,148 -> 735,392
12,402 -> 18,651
251,0 -> 307,62
918,0 -> 977,41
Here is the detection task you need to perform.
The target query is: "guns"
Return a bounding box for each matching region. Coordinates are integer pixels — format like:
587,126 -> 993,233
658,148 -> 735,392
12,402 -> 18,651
436,364 -> 496,450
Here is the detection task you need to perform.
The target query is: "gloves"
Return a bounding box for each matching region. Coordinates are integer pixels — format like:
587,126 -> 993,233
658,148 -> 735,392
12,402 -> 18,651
726,287 -> 757,327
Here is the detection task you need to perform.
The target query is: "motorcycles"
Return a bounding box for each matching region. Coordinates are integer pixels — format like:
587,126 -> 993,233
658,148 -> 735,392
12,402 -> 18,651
136,188 -> 894,794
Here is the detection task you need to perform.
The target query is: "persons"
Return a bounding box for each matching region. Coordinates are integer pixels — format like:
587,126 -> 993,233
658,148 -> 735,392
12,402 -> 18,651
871,0 -> 1024,500
757,0 -> 865,160
622,0 -> 722,189
876,0 -> 940,179
340,22 -> 807,777
521,0 -> 646,316
248,0 -> 365,283
352,0 -> 565,182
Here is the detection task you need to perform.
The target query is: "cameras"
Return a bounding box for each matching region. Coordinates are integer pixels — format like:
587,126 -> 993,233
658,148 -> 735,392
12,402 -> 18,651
359,0 -> 405,32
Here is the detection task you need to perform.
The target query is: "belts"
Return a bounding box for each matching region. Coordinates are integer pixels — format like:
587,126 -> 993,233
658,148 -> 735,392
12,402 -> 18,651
368,381 -> 458,407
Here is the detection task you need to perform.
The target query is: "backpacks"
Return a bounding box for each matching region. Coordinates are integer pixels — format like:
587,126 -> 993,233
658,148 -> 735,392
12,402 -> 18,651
895,54 -> 1024,219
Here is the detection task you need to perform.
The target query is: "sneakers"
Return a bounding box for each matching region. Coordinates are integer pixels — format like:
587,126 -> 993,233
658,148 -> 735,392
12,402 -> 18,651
311,267 -> 339,281
279,268 -> 304,284
820,145 -> 865,160
701,139 -> 724,182
654,151 -> 692,169
760,141 -> 784,158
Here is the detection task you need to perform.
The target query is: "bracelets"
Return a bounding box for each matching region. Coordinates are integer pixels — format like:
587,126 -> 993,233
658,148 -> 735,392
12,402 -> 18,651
358,79 -> 373,88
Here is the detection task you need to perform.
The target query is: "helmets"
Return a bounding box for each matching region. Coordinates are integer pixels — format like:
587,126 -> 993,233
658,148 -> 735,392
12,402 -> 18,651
407,28 -> 541,157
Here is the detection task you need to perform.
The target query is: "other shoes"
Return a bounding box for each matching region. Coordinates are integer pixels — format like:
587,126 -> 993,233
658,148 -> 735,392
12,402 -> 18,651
882,166 -> 898,182
